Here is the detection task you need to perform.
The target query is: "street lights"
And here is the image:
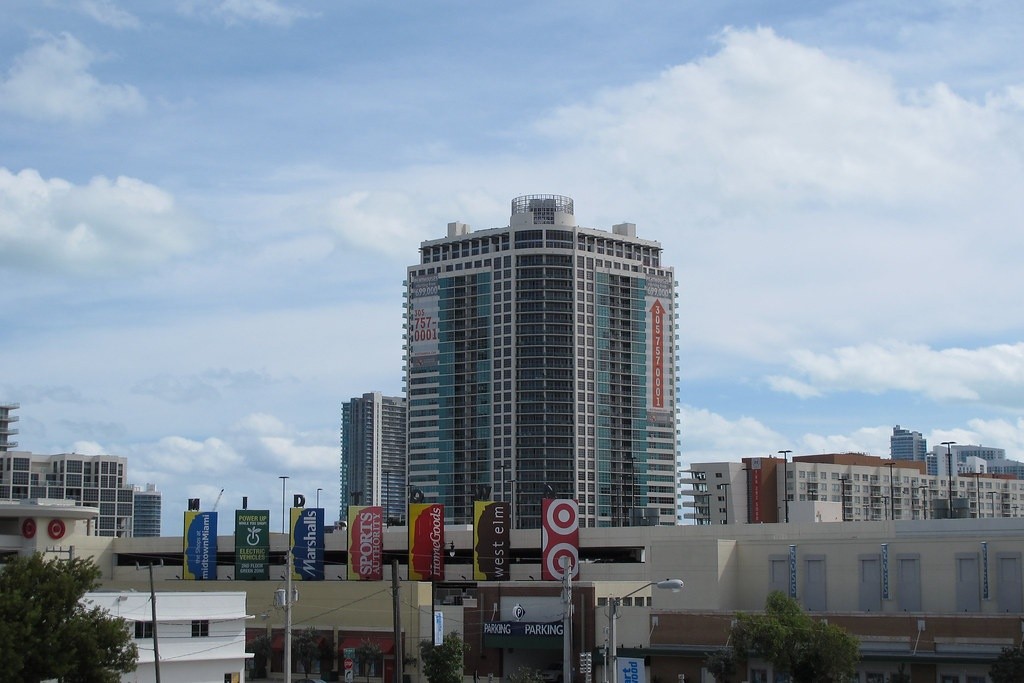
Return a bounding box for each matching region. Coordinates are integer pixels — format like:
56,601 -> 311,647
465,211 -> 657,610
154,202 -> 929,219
987,492 -> 997,517
609,577 -> 687,683
807,489 -> 817,501
777,451 -> 793,522
941,442 -> 956,518
278,476 -> 290,535
693,502 -> 698,525
619,474 -> 632,526
626,456 -> 638,526
742,467 -> 754,524
704,493 -> 712,524
506,479 -> 516,529
431,540 -> 456,648
863,505 -> 870,521
382,471 -> 393,530
316,488 -> 323,508
918,485 -> 928,519
884,462 -> 896,520
498,465 -> 509,501
1011,507 -> 1019,517
972,472 -> 983,518
721,482 -> 731,524
838,478 -> 849,521
881,495 -> 889,520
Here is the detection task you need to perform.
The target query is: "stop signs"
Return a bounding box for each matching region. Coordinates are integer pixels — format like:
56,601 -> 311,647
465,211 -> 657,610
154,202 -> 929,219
343,658 -> 354,670
